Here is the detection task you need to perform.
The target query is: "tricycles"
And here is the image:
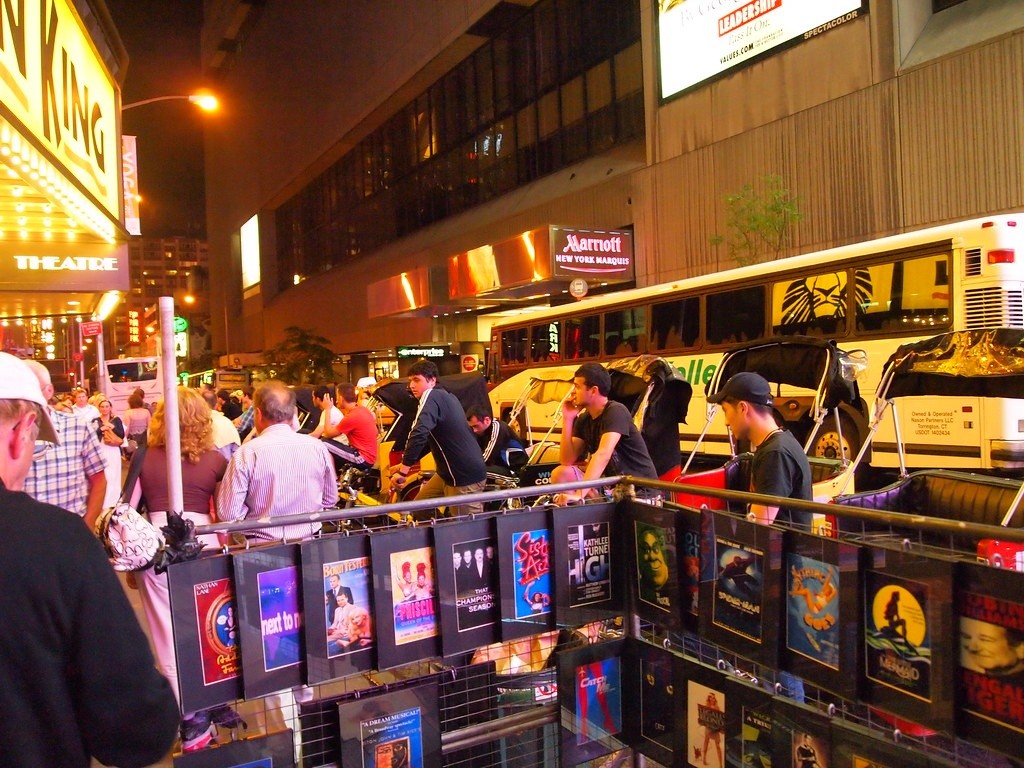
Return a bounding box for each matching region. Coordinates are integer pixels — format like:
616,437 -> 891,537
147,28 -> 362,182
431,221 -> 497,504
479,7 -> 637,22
669,335 -> 873,540
282,354 -> 692,529
825,329 -> 1024,572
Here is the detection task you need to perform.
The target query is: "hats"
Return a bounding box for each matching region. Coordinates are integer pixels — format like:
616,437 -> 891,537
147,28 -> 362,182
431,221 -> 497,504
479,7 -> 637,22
0,348 -> 61,448
707,372 -> 774,407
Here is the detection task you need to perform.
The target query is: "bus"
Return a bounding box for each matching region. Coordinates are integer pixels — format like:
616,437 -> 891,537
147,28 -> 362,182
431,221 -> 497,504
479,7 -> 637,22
212,367 -> 250,397
484,212 -> 1024,478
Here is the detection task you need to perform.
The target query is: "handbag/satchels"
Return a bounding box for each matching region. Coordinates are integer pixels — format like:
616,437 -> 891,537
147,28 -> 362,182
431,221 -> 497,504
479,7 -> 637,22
95,503 -> 168,572
125,440 -> 138,453
103,416 -> 124,447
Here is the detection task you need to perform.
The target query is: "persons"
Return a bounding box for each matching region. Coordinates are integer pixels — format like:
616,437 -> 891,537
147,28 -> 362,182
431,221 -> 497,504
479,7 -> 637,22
702,692 -> 723,768
721,556 -> 761,600
550,363 -> 661,507
395,562 -> 435,602
216,380 -> 339,768
321,383 -> 378,470
136,386 -> 229,738
0,350 -> 180,768
789,564 -> 837,614
199,383 -> 255,435
326,574 -> 373,656
466,404 -> 529,469
707,372 -> 813,532
524,582 -> 550,615
387,360 -> 487,521
454,546 -> 493,591
796,734 -> 822,768
19,359 -> 107,529
49,384 -> 158,509
959,616 -> 1024,681
295,385 -> 349,447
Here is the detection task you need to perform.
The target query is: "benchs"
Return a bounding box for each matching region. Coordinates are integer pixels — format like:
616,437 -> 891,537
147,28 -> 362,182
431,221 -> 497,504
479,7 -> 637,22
831,469 -> 1024,564
670,456 -> 855,537
525,442 -> 560,464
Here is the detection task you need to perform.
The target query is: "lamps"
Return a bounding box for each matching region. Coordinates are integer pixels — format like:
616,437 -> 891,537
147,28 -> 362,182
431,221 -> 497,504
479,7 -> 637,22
122,89 -> 220,113
